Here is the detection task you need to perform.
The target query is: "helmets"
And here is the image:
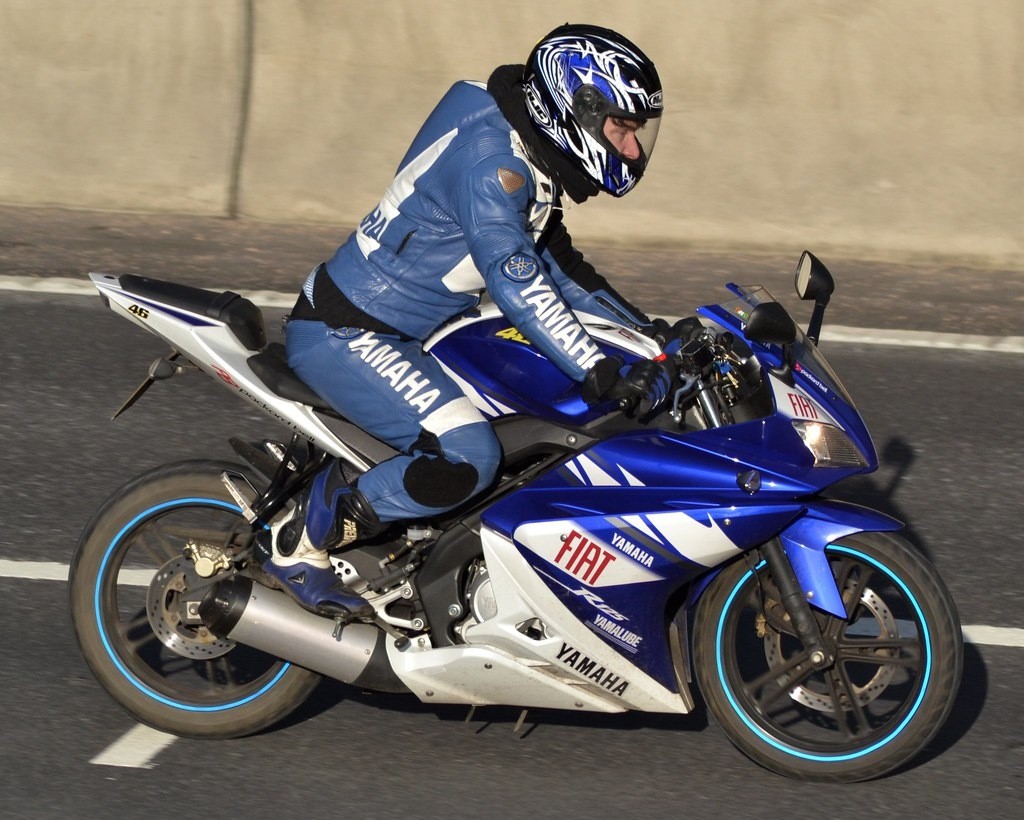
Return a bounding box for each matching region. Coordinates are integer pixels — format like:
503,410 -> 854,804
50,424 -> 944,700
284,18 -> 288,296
525,24 -> 661,199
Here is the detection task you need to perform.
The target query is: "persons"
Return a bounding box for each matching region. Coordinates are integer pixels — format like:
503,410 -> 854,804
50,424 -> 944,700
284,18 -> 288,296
254,23 -> 705,620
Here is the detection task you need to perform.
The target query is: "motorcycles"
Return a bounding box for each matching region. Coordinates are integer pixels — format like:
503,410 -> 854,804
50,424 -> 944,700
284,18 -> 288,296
65,249 -> 966,787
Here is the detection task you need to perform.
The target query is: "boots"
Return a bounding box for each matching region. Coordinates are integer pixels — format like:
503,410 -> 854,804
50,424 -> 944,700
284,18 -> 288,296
247,460 -> 383,617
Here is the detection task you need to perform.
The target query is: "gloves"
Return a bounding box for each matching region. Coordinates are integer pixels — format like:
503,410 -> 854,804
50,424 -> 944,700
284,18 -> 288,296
644,312 -> 709,365
581,356 -> 673,415
